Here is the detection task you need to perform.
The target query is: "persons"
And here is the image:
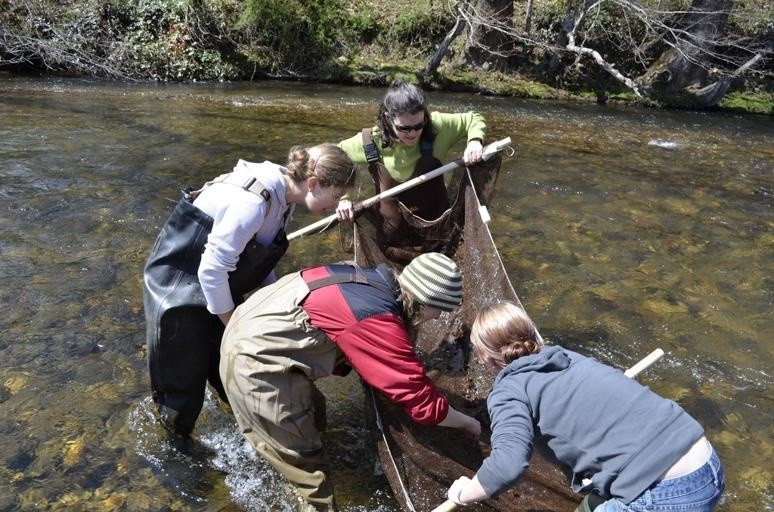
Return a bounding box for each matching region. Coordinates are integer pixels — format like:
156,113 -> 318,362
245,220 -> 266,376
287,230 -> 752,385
140,141 -> 357,460
333,78 -> 489,250
216,249 -> 484,512
443,299 -> 725,512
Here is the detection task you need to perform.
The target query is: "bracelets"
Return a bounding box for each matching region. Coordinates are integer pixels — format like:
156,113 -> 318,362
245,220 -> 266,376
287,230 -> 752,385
457,485 -> 468,504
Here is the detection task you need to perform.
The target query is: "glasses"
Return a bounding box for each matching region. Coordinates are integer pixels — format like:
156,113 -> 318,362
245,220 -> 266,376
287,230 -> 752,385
392,117 -> 428,133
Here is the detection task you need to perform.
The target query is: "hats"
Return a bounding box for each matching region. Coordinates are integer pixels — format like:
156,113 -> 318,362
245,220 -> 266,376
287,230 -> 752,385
397,252 -> 464,312
